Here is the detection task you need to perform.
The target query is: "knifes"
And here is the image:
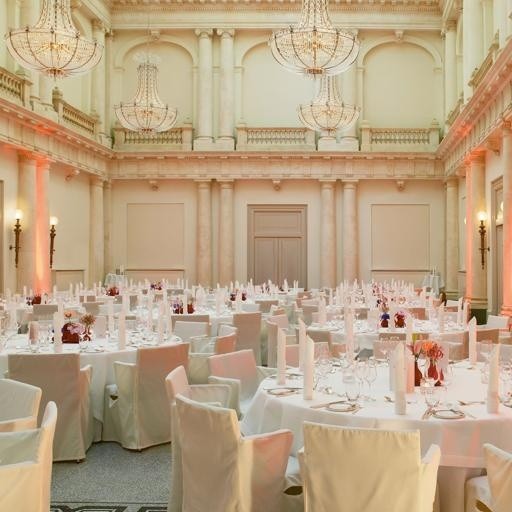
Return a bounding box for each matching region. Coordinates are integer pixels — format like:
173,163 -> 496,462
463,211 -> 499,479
263,387 -> 303,390
310,400 -> 346,409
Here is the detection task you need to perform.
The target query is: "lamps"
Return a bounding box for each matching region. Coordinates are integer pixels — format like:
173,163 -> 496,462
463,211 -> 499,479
50,216 -> 58,268
112,0 -> 179,135
296,75 -> 361,135
3,0 -> 104,80
479,215 -> 489,269
9,212 -> 22,268
268,0 -> 361,80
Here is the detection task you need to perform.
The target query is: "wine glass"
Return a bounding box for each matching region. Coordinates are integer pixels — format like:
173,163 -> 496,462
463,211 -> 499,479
300,336 -> 512,411
327,311 -> 461,333
0,310 -> 163,351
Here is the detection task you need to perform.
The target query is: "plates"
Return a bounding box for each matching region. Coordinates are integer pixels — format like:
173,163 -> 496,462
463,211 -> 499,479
431,407 -> 466,420
265,372 -> 300,397
325,400 -> 359,413
